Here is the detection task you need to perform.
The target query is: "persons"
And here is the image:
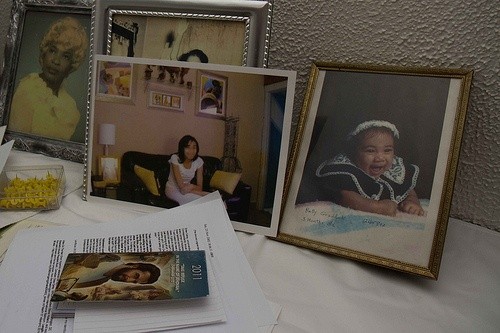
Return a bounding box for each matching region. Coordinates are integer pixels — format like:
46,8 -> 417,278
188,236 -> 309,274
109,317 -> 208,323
165,135 -> 210,206
96,49 -> 223,116
6,16 -> 88,141
54,253 -> 161,301
312,119 -> 424,217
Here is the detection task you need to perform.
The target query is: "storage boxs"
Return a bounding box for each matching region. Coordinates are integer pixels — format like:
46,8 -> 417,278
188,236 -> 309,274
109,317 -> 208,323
264,59 -> 474,282
0,165 -> 67,209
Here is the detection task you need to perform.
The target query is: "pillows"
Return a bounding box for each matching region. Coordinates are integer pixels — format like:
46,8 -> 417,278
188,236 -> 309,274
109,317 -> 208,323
209,171 -> 242,194
134,164 -> 161,196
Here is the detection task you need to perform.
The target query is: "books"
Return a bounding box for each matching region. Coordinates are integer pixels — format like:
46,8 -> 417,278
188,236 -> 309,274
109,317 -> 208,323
50,250 -> 209,318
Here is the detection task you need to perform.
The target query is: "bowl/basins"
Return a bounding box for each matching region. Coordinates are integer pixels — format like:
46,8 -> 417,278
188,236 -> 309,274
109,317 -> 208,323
0,163 -> 67,210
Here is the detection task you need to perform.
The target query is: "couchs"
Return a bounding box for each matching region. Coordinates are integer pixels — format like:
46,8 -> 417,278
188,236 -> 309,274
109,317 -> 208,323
114,150 -> 253,224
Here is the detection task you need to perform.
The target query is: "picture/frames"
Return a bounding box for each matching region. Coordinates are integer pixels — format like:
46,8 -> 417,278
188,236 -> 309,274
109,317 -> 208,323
98,123 -> 116,154
194,69 -> 229,121
0,0 -> 92,163
98,155 -> 121,184
94,59 -> 138,106
81,0 -> 273,203
